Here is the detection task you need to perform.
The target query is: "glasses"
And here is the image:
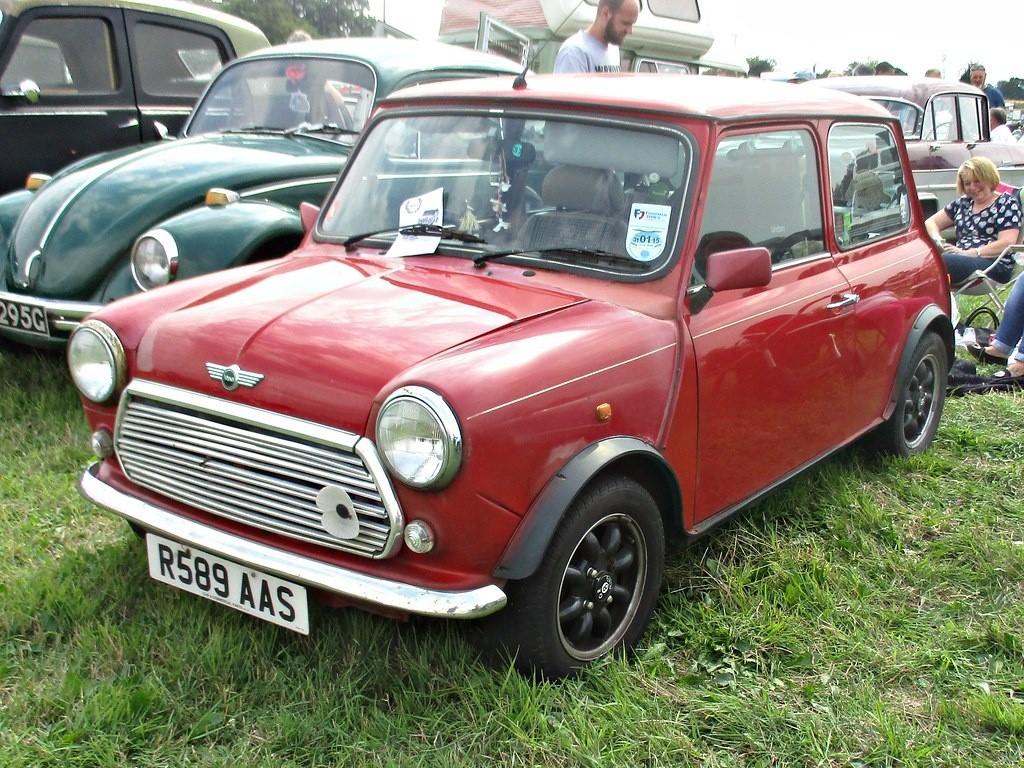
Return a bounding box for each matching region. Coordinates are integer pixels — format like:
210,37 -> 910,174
969,65 -> 986,71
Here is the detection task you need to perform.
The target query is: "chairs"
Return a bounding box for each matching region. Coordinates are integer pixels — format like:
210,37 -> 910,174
951,181 -> 1024,329
514,165 -> 629,260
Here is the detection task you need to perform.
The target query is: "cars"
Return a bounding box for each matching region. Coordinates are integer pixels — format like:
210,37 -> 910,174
67,73 -> 956,677
716,76 -> 1023,239
1,37 -> 538,353
1,1 -> 275,192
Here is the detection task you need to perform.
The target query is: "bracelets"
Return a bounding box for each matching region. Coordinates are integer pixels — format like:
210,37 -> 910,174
975,247 -> 980,257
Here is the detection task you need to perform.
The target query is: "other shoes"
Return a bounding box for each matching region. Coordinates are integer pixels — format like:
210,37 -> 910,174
991,369 -> 1024,383
967,344 -> 1009,366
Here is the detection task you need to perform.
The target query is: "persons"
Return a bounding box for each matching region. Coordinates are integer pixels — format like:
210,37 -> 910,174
925,69 -> 942,79
968,65 -> 1004,107
553,0 -> 639,73
828,62 -> 894,77
989,108 -> 1018,143
967,271 -> 1024,385
923,158 -> 1021,287
263,29 -> 313,135
